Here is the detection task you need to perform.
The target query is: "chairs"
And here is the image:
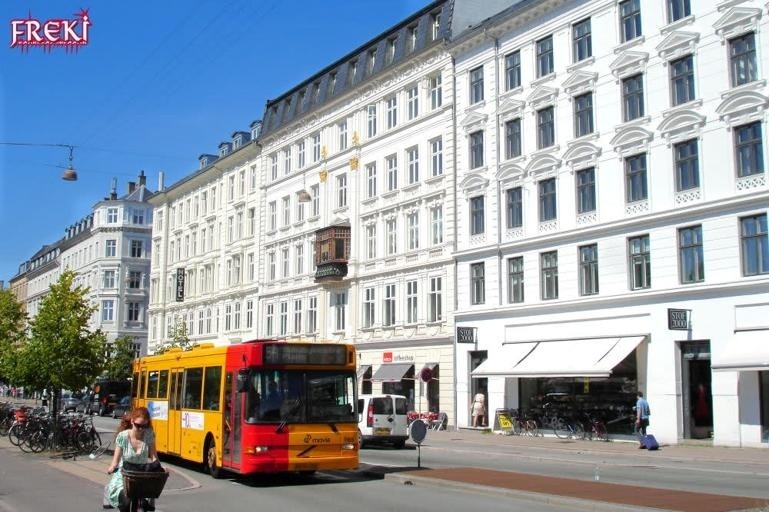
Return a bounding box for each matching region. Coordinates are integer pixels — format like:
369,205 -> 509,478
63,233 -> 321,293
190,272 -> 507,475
432,411 -> 446,431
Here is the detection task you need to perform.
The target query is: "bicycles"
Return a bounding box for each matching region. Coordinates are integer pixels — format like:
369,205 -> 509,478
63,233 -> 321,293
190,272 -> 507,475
0,401 -> 101,454
114,461 -> 169,512
506,404 -> 608,441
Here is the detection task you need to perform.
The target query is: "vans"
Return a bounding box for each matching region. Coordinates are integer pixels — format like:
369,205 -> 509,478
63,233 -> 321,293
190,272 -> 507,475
337,394 -> 409,448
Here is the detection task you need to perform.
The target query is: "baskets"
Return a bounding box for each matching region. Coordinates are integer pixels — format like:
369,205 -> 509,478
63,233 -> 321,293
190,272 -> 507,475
121,465 -> 170,499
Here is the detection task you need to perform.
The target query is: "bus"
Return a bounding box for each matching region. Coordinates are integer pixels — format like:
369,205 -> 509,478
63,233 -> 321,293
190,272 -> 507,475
129,337 -> 360,479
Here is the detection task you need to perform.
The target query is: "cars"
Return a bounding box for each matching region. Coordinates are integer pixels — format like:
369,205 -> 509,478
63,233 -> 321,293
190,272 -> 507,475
62,392 -> 129,418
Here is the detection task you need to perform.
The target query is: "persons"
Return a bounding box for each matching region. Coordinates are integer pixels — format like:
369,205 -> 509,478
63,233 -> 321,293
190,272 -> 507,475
40,388 -> 50,411
264,380 -> 283,401
102,406 -> 170,512
634,390 -> 651,450
5,386 -> 32,399
239,379 -> 260,420
310,388 -> 338,422
471,387 -> 489,429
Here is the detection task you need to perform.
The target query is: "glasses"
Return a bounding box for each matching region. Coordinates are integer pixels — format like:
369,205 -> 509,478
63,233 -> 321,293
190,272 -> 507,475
134,422 -> 149,429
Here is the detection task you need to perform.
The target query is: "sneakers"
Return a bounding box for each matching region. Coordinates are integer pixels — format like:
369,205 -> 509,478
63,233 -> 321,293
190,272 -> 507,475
639,445 -> 645,449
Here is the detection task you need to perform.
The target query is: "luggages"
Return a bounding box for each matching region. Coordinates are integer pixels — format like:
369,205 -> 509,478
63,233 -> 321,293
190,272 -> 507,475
640,435 -> 659,450
